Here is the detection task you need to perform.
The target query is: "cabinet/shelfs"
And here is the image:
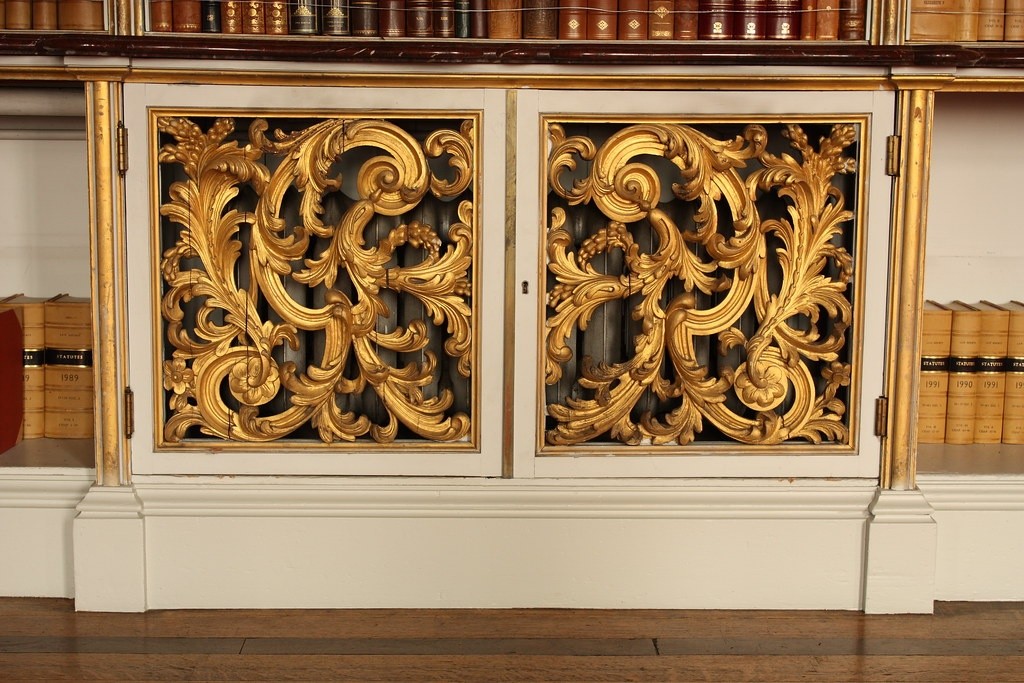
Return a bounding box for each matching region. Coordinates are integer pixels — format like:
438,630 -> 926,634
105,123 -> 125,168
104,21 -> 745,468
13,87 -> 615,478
61,55 -> 938,615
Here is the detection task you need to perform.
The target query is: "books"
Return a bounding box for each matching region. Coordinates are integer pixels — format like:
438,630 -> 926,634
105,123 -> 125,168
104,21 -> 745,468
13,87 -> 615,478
147,0 -> 868,40
0,291 -> 93,452
0,0 -> 104,32
921,299 -> 1023,445
910,0 -> 1024,42
